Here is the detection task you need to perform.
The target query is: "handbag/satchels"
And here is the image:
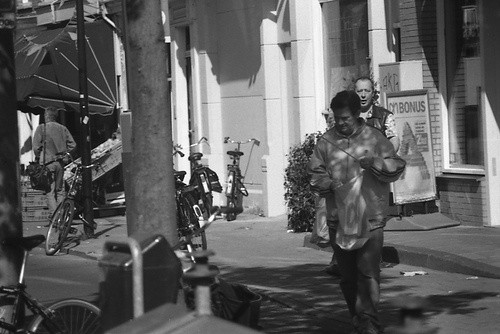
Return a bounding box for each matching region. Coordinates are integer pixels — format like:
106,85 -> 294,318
25,161 -> 54,190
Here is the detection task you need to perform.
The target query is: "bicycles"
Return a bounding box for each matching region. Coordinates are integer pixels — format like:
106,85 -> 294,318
224,137 -> 260,221
0,234 -> 101,334
172,210 -> 262,328
173,144 -> 208,263
45,151 -> 102,256
176,136 -> 223,220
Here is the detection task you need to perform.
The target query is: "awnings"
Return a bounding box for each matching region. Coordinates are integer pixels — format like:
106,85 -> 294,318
15,20 -> 118,116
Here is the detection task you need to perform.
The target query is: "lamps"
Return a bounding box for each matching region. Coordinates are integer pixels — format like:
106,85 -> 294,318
462,6 -> 482,38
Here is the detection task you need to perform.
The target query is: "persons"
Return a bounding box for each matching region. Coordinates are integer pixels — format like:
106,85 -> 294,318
307,90 -> 406,334
33,106 -> 77,226
324,76 -> 401,276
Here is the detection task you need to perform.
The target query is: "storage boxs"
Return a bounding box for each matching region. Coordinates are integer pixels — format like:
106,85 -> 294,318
99,241 -> 182,307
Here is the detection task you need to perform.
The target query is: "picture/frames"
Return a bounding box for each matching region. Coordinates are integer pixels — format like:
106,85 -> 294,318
383,89 -> 438,205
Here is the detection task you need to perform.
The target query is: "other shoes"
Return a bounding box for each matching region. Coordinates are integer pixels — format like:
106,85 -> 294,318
44,223 -> 57,228
